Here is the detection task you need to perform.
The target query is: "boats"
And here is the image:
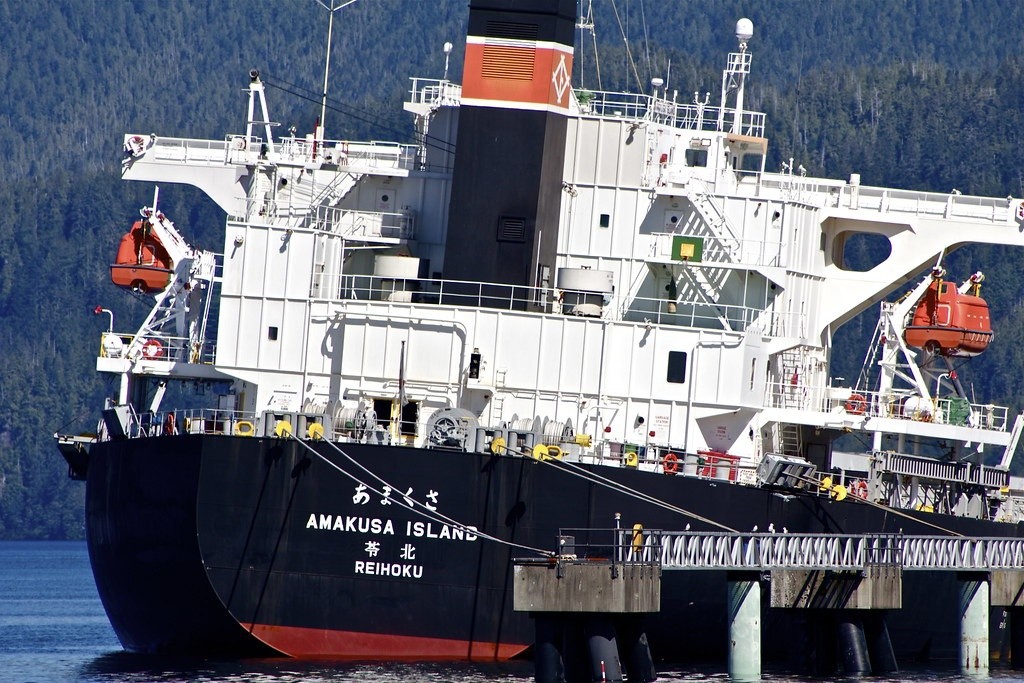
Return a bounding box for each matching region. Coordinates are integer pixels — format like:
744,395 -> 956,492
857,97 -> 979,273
905,281 -> 994,355
52,0 -> 1023,664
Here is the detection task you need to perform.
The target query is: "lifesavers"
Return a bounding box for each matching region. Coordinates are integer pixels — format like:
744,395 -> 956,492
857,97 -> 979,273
142,339 -> 163,360
851,481 -> 869,501
845,394 -> 867,415
664,453 -> 678,475
